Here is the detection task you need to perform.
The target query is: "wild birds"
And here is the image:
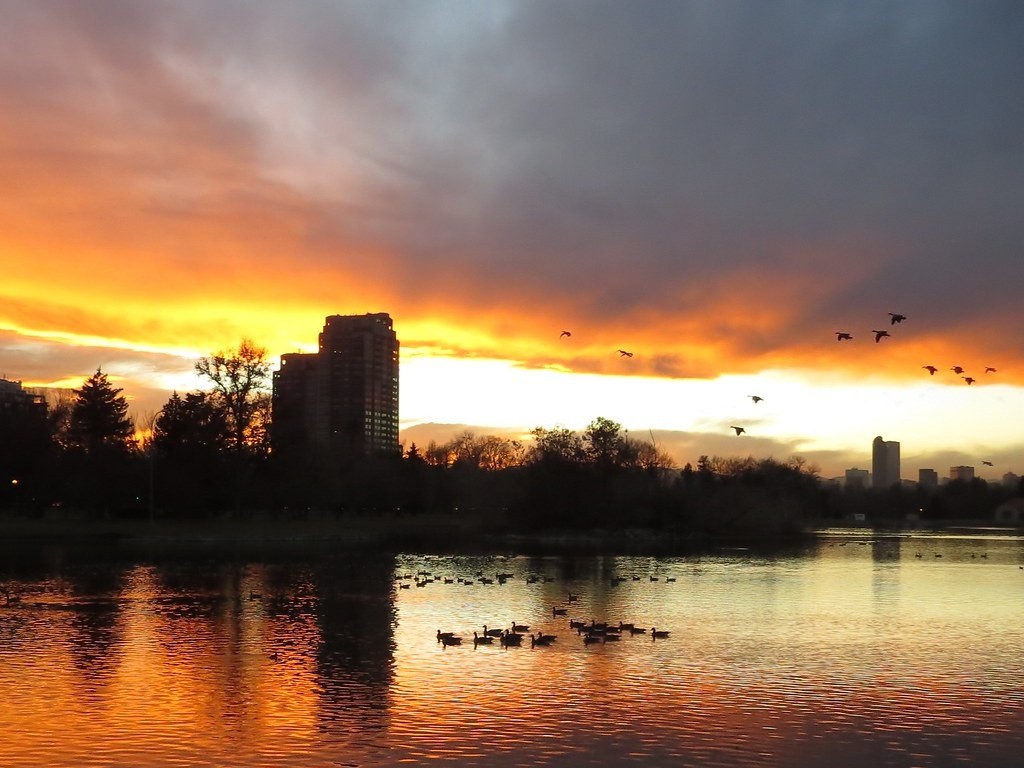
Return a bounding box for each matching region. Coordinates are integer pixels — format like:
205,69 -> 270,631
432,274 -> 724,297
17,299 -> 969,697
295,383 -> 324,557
617,350 -> 633,359
730,426 -> 745,436
922,366 -> 938,375
962,377 -> 976,385
872,330 -> 890,343
247,550 -> 677,619
270,653 -> 278,659
951,365 -> 963,374
437,621 -> 556,645
559,331 -> 570,338
620,621 -> 646,634
835,332 -> 853,341
5,594 -> 18,603
570,620 -> 621,644
748,395 -> 763,404
829,541 -> 989,558
985,366 -> 996,373
651,628 -> 670,636
983,461 -> 993,466
888,312 -> 906,325
